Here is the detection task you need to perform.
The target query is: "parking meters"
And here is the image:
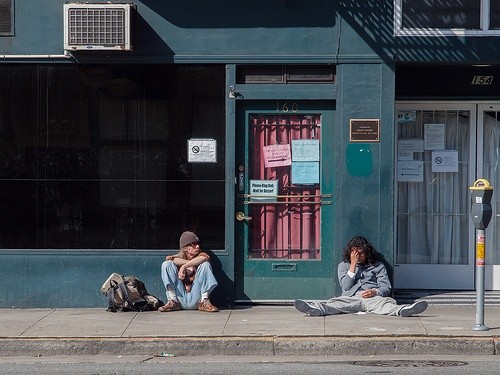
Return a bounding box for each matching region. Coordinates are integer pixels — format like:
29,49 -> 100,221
470,179 -> 494,331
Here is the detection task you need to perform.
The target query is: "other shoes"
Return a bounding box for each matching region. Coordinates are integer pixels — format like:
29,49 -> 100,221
294,299 -> 322,316
399,300 -> 428,317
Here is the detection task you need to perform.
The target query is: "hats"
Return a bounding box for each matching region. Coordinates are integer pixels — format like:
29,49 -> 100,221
180,231 -> 199,248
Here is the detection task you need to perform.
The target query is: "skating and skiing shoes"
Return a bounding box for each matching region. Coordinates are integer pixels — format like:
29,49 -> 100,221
198,298 -> 218,312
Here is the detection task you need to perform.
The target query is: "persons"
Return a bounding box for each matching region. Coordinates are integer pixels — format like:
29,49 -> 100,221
294,236 -> 428,317
159,231 -> 219,312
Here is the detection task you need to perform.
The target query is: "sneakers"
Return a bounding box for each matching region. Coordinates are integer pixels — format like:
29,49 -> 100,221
159,300 -> 180,311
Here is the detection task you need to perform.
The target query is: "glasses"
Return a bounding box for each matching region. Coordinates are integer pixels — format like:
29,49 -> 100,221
186,242 -> 198,248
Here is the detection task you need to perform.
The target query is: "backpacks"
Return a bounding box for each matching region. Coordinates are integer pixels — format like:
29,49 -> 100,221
101,271 -> 159,312
